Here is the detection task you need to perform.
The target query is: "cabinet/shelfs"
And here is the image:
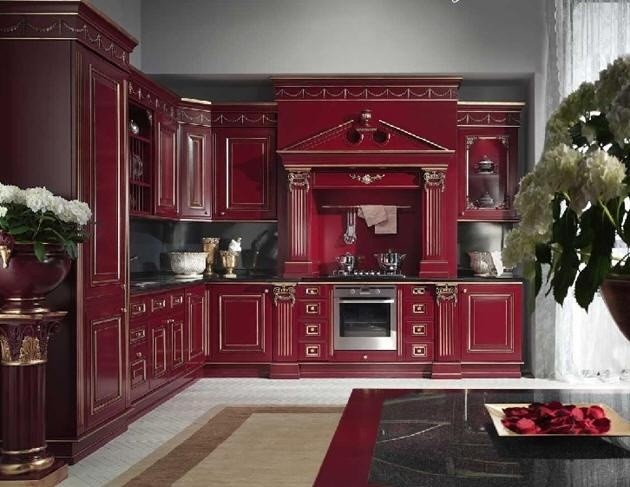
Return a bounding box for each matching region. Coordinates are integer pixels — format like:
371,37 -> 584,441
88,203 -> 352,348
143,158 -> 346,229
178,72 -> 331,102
204,282 -> 273,365
457,284 -> 524,362
129,285 -> 204,402
212,128 -> 277,220
297,284 -> 330,362
128,97 -> 179,218
458,108 -> 522,220
0,42 -> 127,442
402,285 -> 434,362
181,126 -> 212,217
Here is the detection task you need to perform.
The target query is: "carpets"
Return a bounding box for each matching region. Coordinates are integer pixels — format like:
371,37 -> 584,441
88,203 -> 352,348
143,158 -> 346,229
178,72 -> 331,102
102,403 -> 347,487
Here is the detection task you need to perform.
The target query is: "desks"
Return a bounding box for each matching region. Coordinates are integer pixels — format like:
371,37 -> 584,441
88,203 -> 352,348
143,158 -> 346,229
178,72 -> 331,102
311,388 -> 630,487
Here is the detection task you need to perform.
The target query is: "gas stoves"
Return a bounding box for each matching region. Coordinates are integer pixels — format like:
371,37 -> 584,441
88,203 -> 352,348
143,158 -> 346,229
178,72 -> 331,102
300,262 -> 408,281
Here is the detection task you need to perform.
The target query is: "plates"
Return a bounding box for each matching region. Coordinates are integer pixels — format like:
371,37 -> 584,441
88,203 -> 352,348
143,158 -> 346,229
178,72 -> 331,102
482,401 -> 630,440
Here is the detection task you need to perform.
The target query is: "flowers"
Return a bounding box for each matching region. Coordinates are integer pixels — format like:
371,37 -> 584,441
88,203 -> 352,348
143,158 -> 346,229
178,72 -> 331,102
0,182 -> 93,261
501,56 -> 630,314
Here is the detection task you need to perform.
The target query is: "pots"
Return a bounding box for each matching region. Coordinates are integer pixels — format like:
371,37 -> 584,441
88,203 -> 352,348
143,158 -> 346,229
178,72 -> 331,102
372,250 -> 406,272
336,252 -> 362,272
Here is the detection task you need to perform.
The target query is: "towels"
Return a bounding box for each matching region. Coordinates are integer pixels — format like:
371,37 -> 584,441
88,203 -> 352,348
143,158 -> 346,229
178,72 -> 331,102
375,206 -> 397,234
358,205 -> 388,228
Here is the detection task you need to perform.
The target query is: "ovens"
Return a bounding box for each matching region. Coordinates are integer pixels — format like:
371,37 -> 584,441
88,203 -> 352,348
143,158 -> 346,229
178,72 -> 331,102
331,286 -> 398,351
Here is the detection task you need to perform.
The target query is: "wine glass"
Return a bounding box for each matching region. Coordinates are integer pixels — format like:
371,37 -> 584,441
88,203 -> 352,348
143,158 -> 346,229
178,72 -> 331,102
199,238 -> 220,278
220,250 -> 241,279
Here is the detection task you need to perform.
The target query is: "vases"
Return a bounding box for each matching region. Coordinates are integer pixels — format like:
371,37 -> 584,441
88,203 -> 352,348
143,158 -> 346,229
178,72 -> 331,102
0,242 -> 71,314
600,266 -> 630,341
